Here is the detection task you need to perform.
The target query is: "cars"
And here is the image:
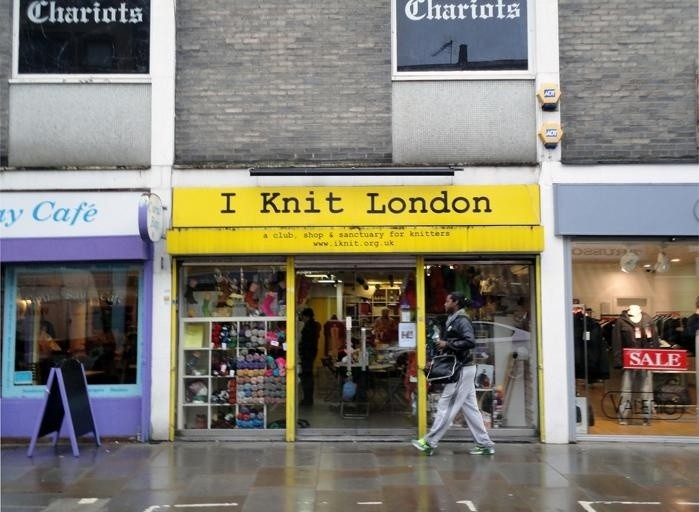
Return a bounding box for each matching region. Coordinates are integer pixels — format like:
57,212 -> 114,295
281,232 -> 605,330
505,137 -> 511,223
387,319 -> 531,374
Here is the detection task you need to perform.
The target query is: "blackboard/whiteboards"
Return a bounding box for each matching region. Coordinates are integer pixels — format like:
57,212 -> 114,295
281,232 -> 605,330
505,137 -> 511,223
56,362 -> 96,438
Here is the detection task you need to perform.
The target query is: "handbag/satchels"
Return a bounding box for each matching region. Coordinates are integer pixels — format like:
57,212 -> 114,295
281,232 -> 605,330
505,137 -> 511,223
425,354 -> 462,385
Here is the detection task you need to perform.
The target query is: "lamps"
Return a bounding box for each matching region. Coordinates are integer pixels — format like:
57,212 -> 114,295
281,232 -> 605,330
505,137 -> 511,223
654,244 -> 670,272
619,243 -> 640,273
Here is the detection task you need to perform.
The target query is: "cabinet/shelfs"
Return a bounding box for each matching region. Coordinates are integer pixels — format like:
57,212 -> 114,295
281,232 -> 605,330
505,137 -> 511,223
176,261 -> 402,435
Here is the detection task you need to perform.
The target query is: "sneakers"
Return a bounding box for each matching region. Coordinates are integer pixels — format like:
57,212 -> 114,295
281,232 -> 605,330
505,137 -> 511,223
411,437 -> 434,456
466,445 -> 496,455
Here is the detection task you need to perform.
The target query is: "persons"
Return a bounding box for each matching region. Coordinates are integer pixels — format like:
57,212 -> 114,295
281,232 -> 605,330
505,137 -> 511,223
408,291 -> 497,455
296,302 -> 322,410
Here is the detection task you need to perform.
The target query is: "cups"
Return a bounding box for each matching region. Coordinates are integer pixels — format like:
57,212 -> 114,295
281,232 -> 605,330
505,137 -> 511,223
432,335 -> 440,341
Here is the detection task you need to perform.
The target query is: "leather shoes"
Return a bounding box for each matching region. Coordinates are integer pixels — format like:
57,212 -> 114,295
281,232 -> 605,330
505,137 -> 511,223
299,397 -> 314,407
